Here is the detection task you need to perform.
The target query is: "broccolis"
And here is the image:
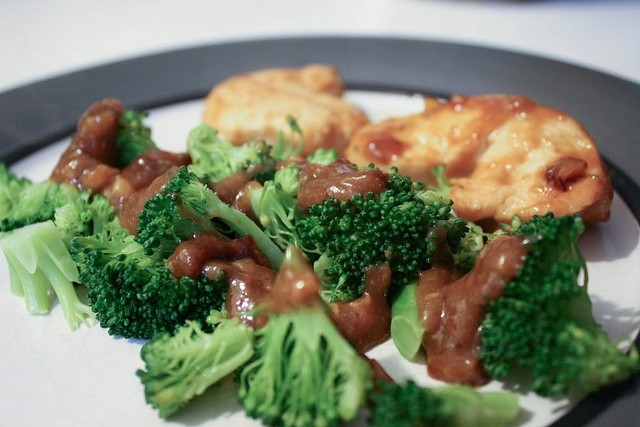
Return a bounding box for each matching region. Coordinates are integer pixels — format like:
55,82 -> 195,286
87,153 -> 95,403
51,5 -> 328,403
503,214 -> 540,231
0,100 -> 640,427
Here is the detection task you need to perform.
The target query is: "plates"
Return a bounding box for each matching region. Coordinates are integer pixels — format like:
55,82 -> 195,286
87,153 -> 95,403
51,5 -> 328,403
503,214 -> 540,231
0,34 -> 639,426
